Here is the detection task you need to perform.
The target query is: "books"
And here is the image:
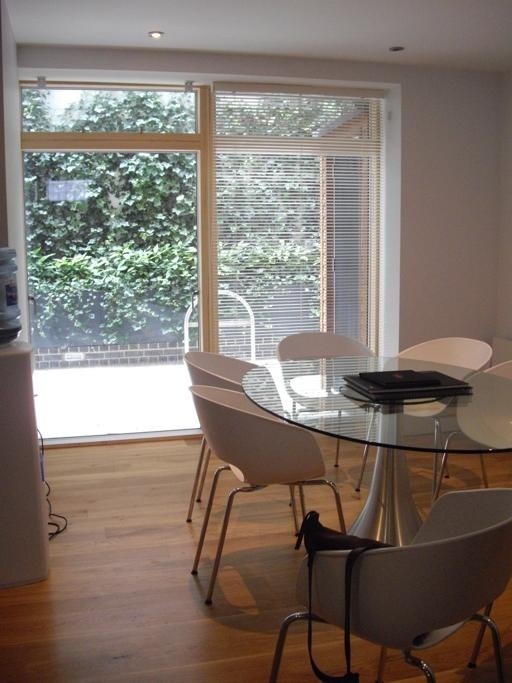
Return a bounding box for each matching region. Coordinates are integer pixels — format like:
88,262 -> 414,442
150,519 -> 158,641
342,370 -> 469,394
345,382 -> 475,401
357,369 -> 442,388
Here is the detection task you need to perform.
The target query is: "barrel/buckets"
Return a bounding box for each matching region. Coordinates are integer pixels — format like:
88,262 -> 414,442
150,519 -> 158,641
0,246 -> 23,342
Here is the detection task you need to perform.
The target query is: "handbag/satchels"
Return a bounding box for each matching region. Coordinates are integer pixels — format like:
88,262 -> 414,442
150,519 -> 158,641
292,508 -> 430,683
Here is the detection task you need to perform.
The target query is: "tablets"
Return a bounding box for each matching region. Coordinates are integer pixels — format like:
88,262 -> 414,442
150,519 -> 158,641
343,371 -> 473,403
